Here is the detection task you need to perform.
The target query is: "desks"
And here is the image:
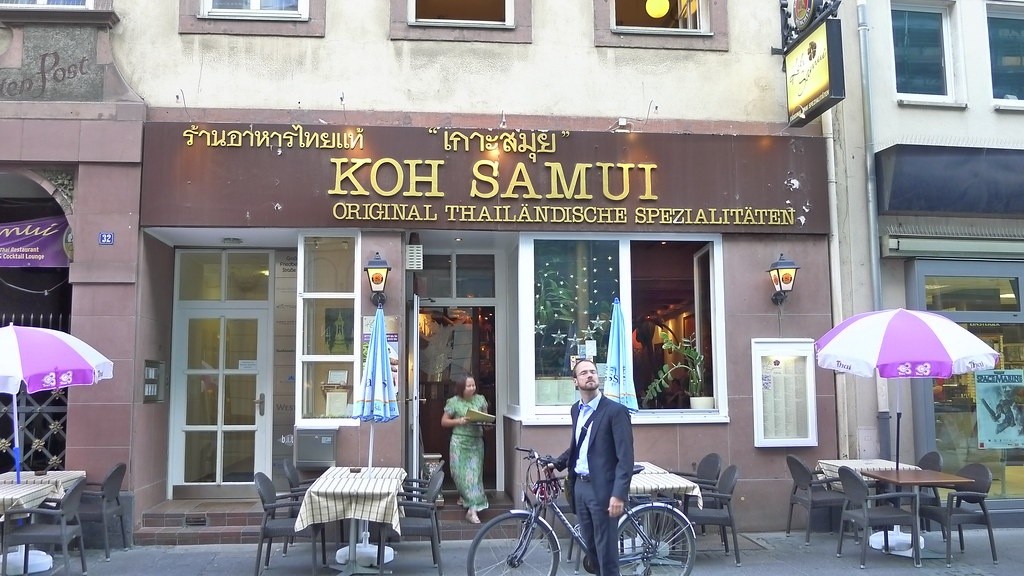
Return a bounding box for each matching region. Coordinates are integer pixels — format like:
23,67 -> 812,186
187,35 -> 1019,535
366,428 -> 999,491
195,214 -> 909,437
294,478 -> 406,576
622,473 -> 703,576
815,459 -> 923,537
315,466 -> 407,543
617,462 -> 670,541
0,470 -> 86,548
0,483 -> 56,576
861,469 -> 975,560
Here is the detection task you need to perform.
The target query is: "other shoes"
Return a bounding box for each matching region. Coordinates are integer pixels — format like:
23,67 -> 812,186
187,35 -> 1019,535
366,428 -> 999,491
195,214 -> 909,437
457,496 -> 464,504
466,512 -> 480,523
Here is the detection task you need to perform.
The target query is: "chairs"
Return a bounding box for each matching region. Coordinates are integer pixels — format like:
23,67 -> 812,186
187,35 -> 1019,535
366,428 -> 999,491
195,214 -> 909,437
545,452 -> 739,574
786,451 -> 1000,567
1,463 -> 127,576
253,460 -> 446,576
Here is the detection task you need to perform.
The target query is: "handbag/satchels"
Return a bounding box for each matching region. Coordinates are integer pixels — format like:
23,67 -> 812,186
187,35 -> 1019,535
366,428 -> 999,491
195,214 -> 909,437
564,476 -> 575,503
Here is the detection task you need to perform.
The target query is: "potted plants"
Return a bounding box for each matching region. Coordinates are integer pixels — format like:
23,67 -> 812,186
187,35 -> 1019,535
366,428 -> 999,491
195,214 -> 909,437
641,333 -> 715,409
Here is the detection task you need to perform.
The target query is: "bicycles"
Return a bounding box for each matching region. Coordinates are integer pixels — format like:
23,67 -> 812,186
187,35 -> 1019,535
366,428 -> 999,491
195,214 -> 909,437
459,446 -> 698,576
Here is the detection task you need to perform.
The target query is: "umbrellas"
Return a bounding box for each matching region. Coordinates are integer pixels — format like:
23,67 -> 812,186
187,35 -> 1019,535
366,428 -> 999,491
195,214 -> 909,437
0,323 -> 114,556
814,308 -> 1000,536
336,304 -> 400,548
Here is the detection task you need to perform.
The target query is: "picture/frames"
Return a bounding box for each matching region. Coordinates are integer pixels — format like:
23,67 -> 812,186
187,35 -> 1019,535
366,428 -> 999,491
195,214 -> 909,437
683,312 -> 711,372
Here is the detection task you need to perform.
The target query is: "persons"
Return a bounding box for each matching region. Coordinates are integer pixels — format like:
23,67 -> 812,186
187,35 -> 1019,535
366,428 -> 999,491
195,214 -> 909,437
441,373 -> 493,524
542,358 -> 634,576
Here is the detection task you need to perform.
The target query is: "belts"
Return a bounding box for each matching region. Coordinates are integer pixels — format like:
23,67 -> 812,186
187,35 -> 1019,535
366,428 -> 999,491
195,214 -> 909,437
577,474 -> 591,482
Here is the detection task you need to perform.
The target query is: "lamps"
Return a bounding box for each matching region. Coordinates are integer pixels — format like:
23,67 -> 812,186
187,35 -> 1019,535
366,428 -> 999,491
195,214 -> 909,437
765,254 -> 802,306
645,0 -> 670,18
365,252 -> 392,307
